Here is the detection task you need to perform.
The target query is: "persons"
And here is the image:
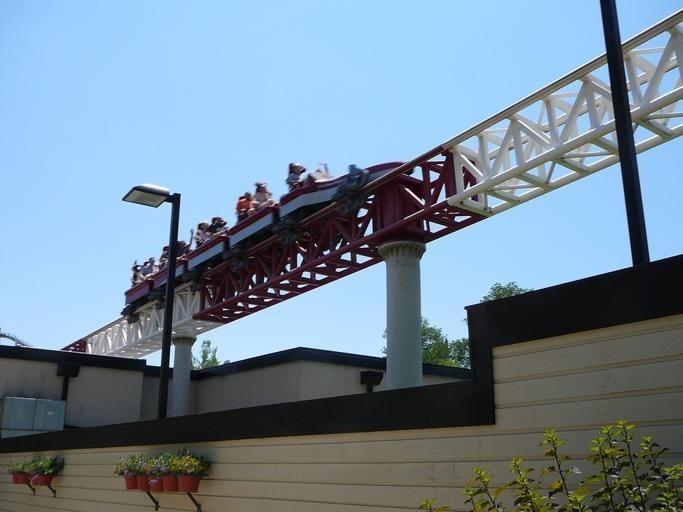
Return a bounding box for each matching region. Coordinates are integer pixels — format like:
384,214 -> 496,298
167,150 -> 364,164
129,161 -> 327,284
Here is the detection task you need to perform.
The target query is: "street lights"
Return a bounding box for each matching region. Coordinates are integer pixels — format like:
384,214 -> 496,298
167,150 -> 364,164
122,183 -> 182,419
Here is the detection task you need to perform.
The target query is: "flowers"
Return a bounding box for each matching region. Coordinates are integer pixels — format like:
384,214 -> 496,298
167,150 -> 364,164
112,445 -> 210,486
5,455 -> 66,478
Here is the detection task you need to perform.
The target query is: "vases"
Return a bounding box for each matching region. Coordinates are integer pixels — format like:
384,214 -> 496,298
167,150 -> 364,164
124,475 -> 201,493
12,473 -> 54,486
